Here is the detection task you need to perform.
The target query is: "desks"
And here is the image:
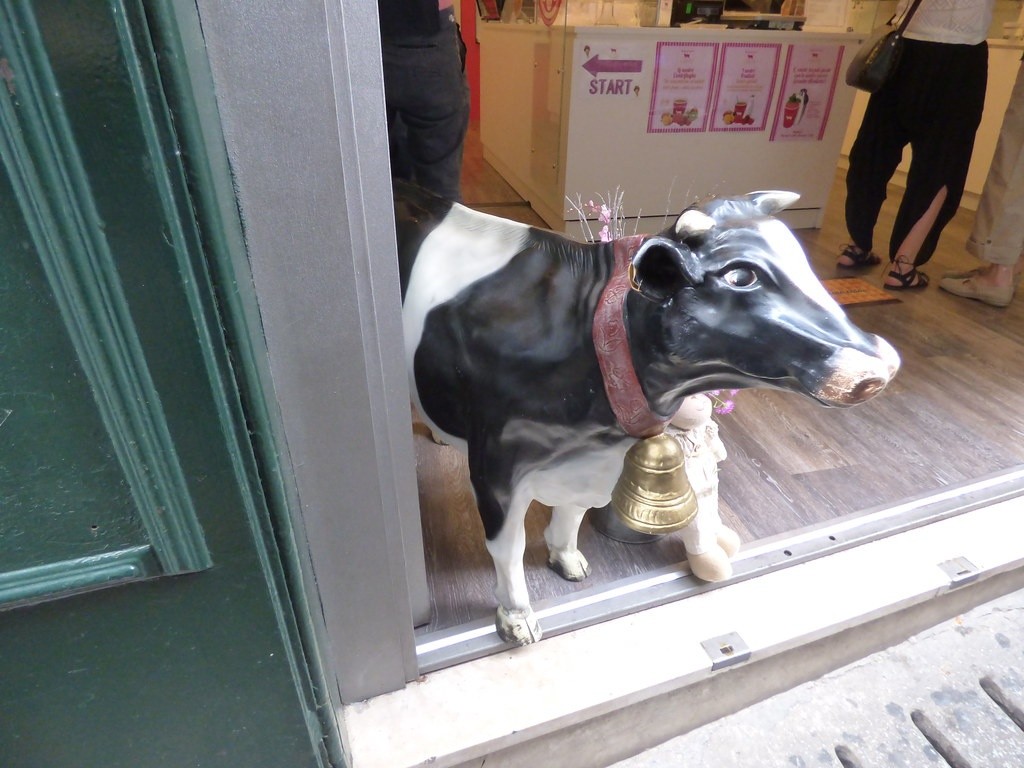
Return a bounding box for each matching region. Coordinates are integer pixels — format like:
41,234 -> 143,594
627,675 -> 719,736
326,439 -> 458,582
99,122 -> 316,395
479,22 -> 869,240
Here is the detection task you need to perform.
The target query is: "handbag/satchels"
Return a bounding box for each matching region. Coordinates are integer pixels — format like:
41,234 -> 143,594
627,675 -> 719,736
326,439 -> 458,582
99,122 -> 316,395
845,24 -> 901,95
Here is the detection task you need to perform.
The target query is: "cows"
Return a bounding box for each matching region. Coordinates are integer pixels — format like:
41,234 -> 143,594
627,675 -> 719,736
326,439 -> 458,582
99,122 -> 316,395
390,176 -> 901,645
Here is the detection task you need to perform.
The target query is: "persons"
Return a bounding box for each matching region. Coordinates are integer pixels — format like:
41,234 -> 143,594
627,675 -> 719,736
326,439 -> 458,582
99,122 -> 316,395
838,0 -> 990,290
376,0 -> 471,305
940,54 -> 1024,308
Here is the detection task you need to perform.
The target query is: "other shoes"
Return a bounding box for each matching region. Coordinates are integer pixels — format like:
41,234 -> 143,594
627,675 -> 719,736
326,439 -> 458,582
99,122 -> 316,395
941,269 -> 1024,300
938,276 -> 1014,308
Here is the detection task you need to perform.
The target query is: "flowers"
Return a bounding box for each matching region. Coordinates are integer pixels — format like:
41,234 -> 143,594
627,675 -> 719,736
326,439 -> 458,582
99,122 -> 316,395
559,185 -> 642,243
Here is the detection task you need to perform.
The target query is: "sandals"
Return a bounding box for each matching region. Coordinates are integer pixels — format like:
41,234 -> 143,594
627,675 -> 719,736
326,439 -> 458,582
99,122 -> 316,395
882,255 -> 930,291
834,242 -> 882,278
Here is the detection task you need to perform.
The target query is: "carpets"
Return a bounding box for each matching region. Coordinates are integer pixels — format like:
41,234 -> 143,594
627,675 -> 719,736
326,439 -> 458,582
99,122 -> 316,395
822,276 -> 904,309
